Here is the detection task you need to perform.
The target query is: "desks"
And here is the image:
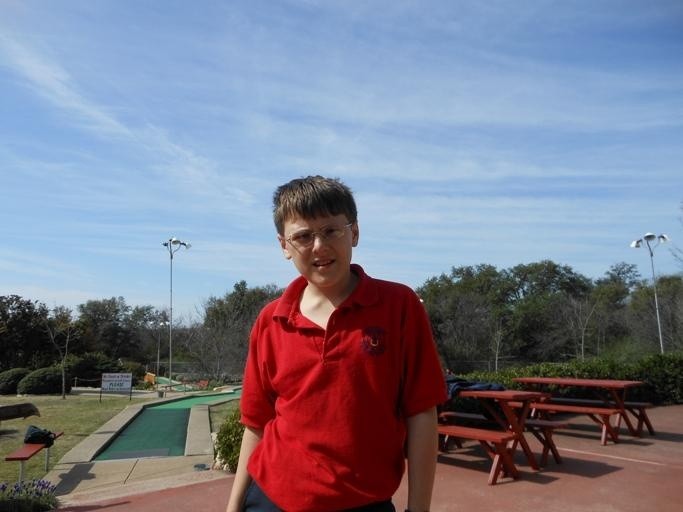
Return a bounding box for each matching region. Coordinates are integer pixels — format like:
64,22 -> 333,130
452,378 -> 645,474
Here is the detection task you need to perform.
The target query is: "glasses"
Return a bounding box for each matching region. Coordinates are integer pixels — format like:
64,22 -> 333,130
282,222 -> 352,248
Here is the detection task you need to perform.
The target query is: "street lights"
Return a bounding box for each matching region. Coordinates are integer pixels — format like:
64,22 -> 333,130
161,237 -> 191,384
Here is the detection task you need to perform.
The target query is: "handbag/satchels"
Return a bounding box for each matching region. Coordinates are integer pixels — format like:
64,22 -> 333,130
24,425 -> 55,448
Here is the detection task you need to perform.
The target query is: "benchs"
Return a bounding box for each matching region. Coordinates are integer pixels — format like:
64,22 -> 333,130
442,403 -> 656,484
4,432 -> 65,487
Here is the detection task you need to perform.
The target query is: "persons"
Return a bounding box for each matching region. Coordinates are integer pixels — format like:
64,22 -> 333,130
225,175 -> 449,512
